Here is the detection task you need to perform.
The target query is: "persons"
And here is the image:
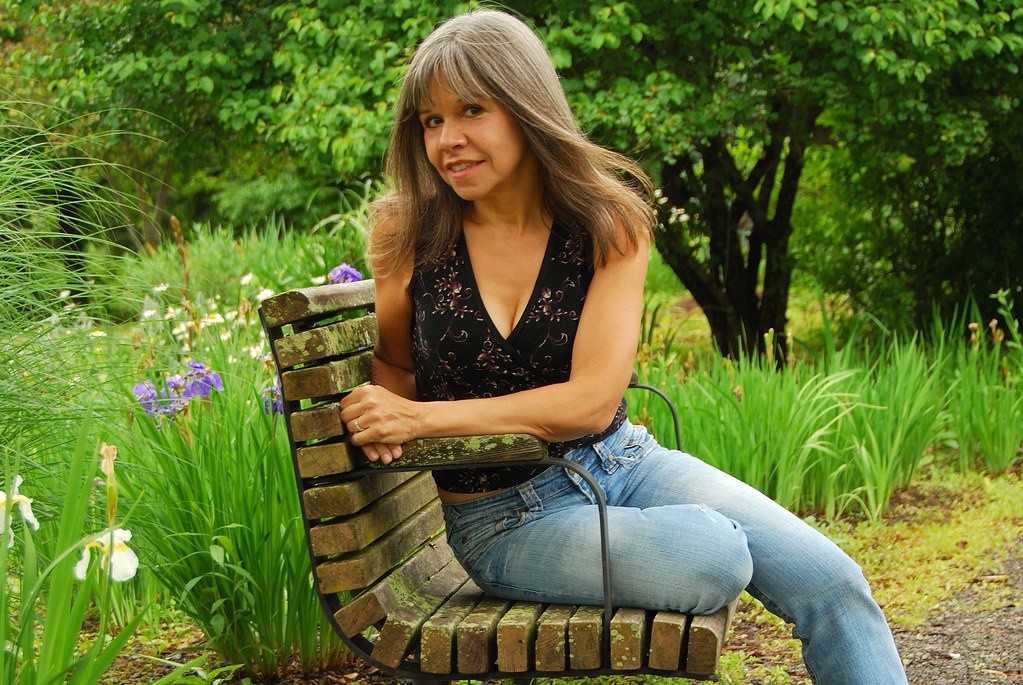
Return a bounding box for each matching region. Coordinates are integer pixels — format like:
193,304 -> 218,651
338,10 -> 909,685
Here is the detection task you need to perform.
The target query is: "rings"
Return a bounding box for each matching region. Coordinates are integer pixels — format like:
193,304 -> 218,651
355,421 -> 365,431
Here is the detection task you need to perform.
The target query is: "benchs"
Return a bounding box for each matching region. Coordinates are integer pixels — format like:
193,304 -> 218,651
257,271 -> 741,685
